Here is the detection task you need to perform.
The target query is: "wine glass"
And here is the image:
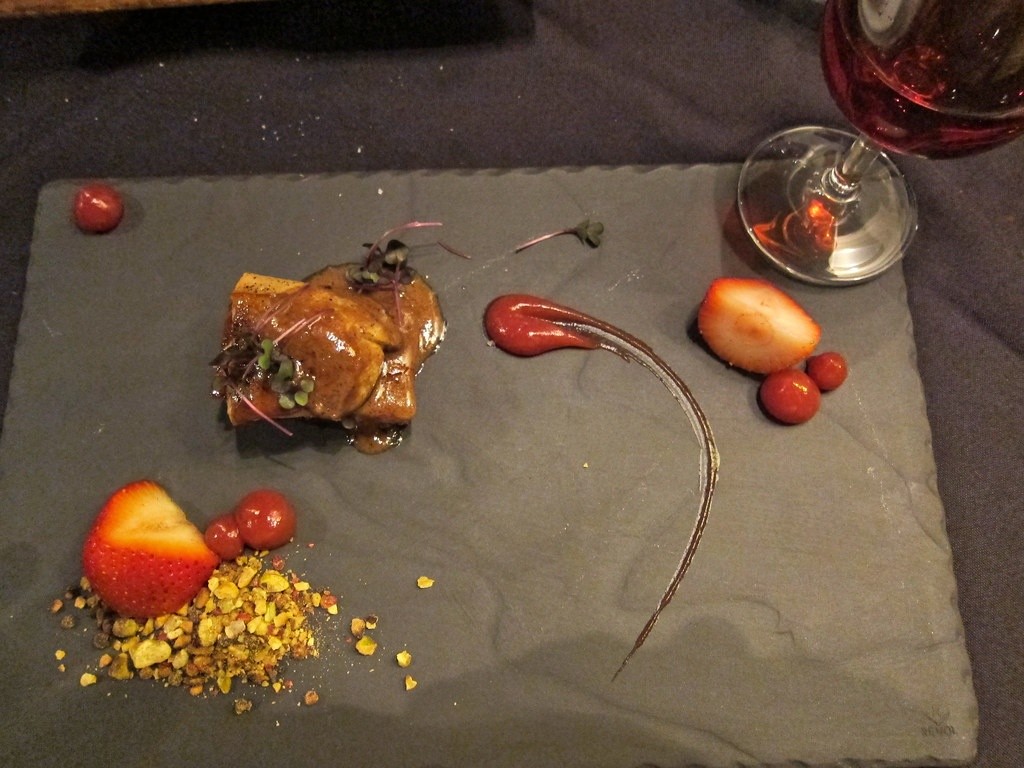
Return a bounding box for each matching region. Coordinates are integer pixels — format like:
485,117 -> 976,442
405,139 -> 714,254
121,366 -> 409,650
737,1 -> 1023,288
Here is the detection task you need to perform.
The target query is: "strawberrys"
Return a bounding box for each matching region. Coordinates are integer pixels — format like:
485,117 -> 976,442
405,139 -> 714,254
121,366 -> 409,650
697,277 -> 822,375
83,478 -> 220,618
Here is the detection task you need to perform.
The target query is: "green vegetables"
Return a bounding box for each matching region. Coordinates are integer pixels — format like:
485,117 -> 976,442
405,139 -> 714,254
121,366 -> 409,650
209,216 -> 606,438
